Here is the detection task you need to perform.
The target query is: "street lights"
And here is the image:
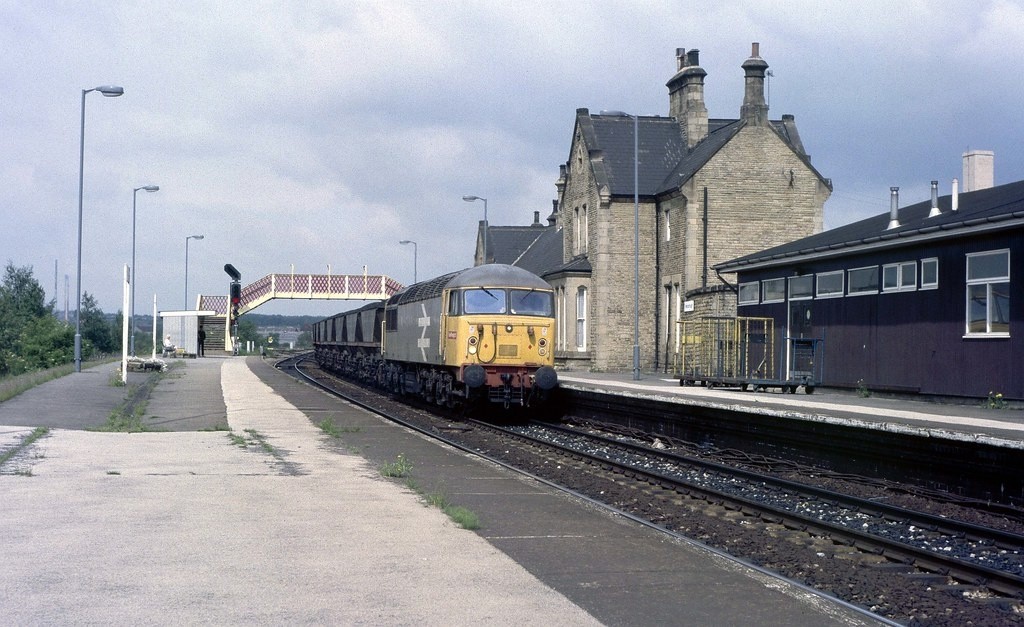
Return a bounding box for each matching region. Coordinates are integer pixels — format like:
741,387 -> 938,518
463,194 -> 488,265
600,109 -> 640,382
130,185 -> 160,359
399,240 -> 418,284
184,234 -> 204,311
74,85 -> 124,375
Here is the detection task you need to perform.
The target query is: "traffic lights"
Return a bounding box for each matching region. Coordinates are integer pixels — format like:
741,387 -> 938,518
230,282 -> 242,304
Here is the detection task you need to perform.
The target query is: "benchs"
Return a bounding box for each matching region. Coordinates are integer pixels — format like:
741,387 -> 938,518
160,340 -> 177,357
183,352 -> 197,359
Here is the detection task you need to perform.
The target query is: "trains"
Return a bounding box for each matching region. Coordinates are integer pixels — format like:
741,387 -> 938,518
313,263 -> 558,420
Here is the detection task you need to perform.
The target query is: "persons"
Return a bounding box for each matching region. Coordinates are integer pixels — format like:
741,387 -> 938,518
198,325 -> 206,358
165,334 -> 178,358
